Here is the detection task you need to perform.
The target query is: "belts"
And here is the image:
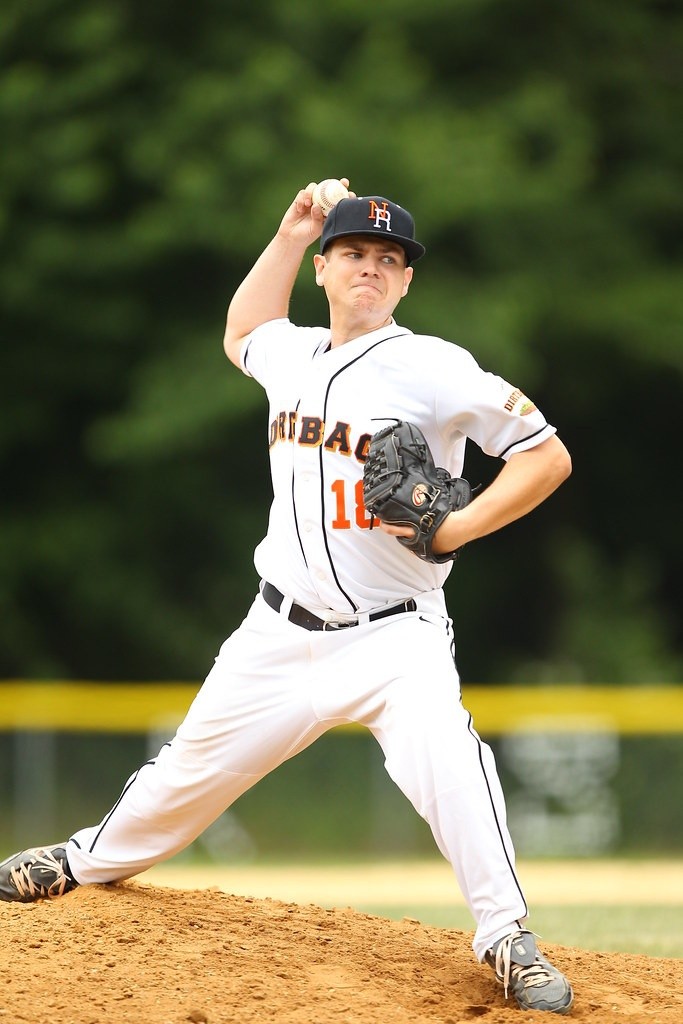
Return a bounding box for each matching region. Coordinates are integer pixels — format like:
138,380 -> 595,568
262,582 -> 417,631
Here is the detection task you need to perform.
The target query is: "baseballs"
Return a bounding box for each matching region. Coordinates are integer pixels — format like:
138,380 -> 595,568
311,177 -> 350,217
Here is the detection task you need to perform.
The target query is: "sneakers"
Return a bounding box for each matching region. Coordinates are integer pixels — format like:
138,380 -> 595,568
485,928 -> 574,1015
0,842 -> 79,902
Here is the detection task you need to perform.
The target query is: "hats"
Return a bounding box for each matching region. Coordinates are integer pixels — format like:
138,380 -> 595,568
320,196 -> 425,267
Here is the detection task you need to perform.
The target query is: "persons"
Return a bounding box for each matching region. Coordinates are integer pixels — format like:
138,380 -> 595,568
0,179 -> 577,1013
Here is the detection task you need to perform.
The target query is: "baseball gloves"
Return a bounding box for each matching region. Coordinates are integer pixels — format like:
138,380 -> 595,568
359,422 -> 474,563
